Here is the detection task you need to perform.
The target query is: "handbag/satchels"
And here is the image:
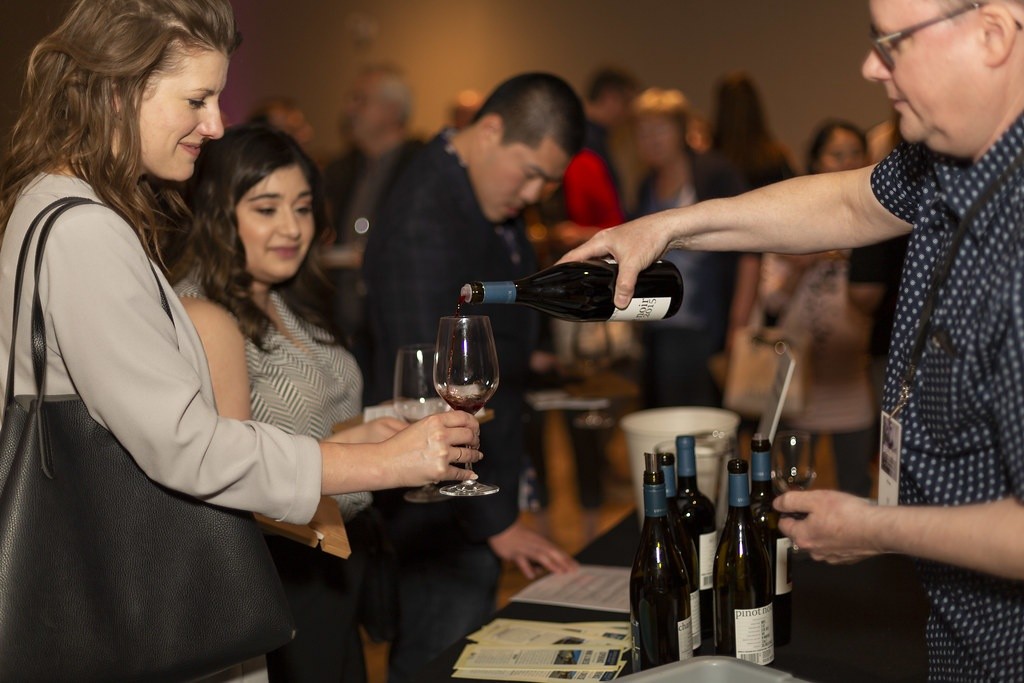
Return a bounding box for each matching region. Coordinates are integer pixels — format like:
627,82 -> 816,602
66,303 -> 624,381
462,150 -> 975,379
0,198 -> 296,683
727,328 -> 803,414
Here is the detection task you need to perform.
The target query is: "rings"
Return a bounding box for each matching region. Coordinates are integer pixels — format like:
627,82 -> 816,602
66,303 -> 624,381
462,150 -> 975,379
456,448 -> 462,462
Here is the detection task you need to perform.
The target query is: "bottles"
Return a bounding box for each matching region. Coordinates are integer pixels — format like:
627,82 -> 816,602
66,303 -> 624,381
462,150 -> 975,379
630,434 -> 794,668
462,257 -> 684,321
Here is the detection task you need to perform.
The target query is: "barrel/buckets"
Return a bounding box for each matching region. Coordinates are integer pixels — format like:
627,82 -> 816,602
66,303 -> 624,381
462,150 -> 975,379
623,407 -> 739,531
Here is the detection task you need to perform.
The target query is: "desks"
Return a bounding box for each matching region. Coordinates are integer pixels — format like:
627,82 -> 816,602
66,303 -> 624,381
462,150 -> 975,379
415,505 -> 930,683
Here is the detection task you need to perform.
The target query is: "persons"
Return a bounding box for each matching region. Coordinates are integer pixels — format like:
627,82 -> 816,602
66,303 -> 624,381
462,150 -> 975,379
0,0 -> 483,683
248,58 -> 911,522
355,72 -> 588,683
178,118 -> 417,683
553,0 -> 1024,682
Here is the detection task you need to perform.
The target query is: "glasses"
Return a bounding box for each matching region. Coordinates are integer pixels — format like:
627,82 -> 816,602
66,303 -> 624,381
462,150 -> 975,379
871,0 -> 1022,71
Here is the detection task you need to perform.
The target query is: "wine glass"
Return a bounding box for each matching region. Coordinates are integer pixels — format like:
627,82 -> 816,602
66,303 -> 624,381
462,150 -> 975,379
393,344 -> 451,501
570,323 -> 613,427
432,316 -> 499,497
